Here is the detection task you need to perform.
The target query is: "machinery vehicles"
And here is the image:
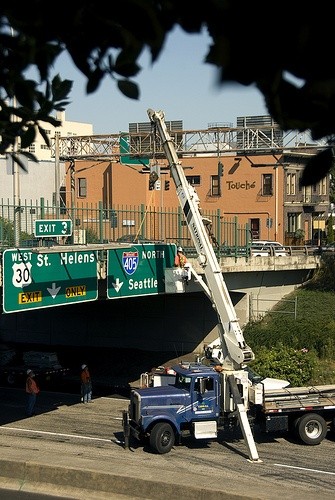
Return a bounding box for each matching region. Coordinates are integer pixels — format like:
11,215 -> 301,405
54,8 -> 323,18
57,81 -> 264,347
122,108 -> 335,463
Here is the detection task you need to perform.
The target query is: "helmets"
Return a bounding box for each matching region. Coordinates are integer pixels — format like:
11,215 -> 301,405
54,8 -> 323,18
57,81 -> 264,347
177,246 -> 183,252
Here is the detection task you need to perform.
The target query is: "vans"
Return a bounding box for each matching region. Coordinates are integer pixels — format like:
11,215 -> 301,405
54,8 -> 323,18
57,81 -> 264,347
248,242 -> 286,257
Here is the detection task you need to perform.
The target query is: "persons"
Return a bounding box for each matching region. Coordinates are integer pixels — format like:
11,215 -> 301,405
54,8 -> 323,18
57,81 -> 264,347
24,369 -> 39,416
81,365 -> 94,404
172,247 -> 188,268
